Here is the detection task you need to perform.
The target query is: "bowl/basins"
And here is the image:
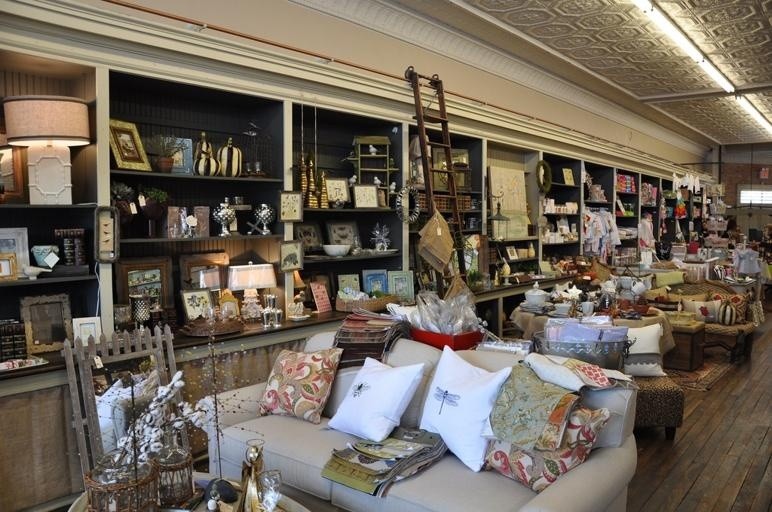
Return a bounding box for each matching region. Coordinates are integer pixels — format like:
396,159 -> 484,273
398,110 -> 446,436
523,292 -> 548,304
321,244 -> 350,257
555,304 -> 572,314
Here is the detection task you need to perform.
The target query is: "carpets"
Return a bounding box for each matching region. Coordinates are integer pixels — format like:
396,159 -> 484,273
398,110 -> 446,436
664,343 -> 746,392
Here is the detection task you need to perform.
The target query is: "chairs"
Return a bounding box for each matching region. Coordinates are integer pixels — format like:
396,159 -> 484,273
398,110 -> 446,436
595,314 -> 685,444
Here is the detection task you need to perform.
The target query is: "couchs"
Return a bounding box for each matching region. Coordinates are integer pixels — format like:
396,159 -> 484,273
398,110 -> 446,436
650,279 -> 765,364
191,332 -> 637,511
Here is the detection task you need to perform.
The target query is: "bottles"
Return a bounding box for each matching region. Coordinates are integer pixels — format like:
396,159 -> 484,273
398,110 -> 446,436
528,242 -> 536,258
260,294 -> 283,328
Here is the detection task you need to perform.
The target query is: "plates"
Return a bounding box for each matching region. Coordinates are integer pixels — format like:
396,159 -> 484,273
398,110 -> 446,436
362,247 -> 400,256
547,309 -> 572,318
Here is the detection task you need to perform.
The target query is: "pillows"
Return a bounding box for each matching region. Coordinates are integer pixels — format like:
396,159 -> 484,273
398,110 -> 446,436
642,285 -> 751,327
619,322 -> 667,379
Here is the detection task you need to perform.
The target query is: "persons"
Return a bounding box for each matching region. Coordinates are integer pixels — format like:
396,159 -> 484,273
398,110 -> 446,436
754,223 -> 772,312
636,210 -> 658,266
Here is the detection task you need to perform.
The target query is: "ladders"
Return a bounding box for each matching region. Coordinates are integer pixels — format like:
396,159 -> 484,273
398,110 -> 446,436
405,65 -> 468,300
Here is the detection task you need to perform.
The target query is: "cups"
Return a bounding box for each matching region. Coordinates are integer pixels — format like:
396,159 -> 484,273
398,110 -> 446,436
581,302 -> 594,316
617,276 -> 652,298
245,160 -> 263,174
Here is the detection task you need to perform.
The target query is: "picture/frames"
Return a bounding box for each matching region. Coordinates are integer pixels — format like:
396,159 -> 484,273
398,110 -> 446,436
19,294 -> 74,355
